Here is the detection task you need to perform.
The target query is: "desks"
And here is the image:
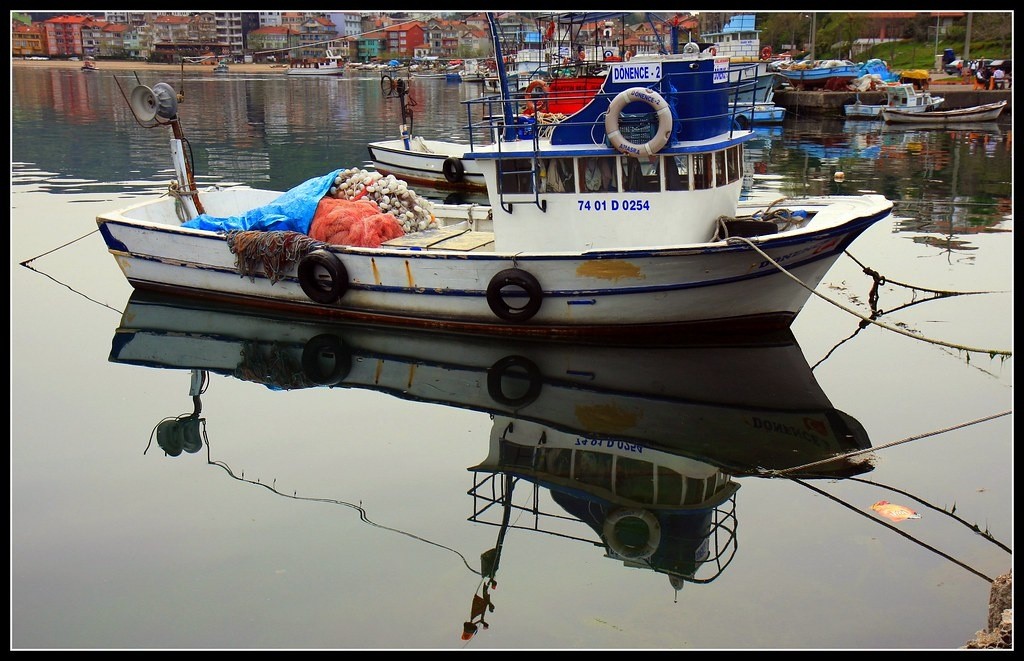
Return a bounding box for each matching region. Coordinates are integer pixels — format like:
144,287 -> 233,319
381,229 -> 494,252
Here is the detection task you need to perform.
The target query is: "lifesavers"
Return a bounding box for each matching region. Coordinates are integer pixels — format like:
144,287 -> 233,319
734,116 -> 748,130
719,221 -> 778,239
488,356 -> 542,405
298,250 -> 348,305
443,158 -> 463,182
888,82 -> 900,86
487,269 -> 543,322
302,335 -> 350,385
605,87 -> 672,157
663,22 -> 671,34
605,510 -> 661,561
525,80 -> 549,111
762,48 -> 771,60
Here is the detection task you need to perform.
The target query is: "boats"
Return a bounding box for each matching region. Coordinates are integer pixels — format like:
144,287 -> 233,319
107,289 -> 874,640
93,12 -> 894,336
80,60 -> 99,72
347,60 -> 517,94
286,56 -> 344,75
368,79 -> 489,194
214,64 -> 228,73
762,57 -> 1008,124
726,54 -> 1010,142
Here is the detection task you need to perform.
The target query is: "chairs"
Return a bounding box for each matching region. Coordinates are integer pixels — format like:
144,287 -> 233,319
973,75 -> 985,90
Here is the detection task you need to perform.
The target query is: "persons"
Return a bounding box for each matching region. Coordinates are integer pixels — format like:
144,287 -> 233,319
957,56 -> 1012,90
